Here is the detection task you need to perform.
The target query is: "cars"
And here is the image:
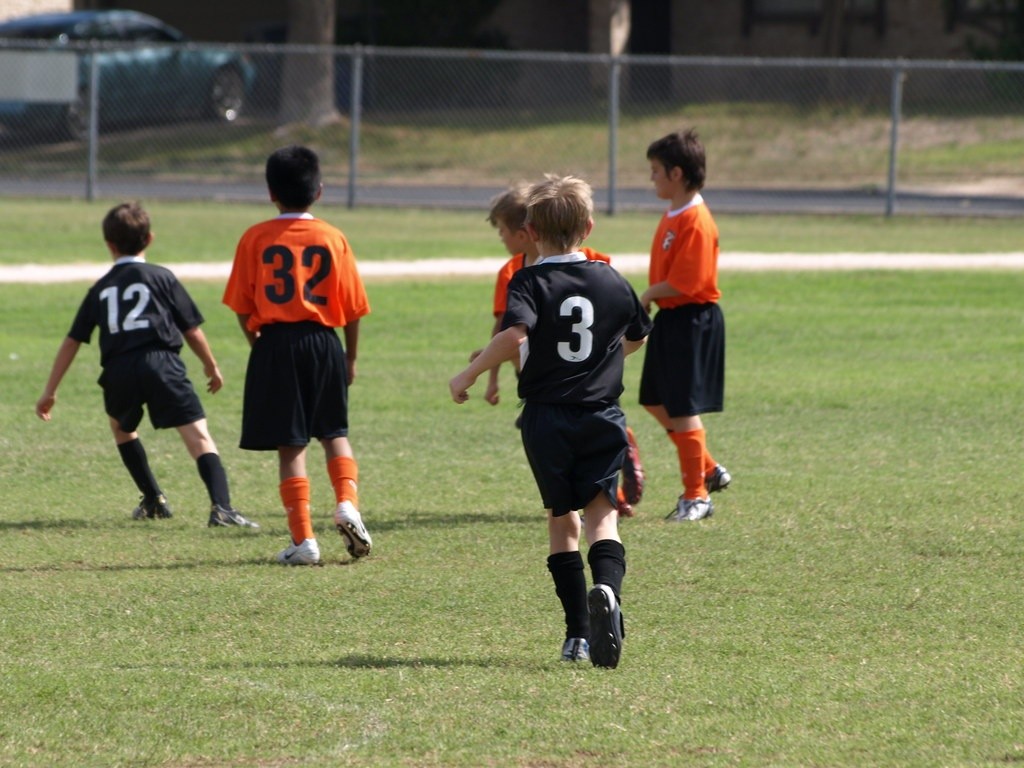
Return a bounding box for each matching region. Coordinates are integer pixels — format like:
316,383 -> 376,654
1,12 -> 258,142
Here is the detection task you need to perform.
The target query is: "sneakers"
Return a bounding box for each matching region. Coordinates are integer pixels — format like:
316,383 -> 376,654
335,501 -> 371,558
619,503 -> 634,517
621,445 -> 643,503
561,637 -> 590,661
665,495 -> 713,520
208,504 -> 258,528
133,494 -> 173,519
279,538 -> 320,565
587,583 -> 624,669
705,464 -> 731,493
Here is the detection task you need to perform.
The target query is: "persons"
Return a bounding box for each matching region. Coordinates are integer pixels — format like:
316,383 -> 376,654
485,183 -> 645,517
37,203 -> 258,529
640,131 -> 729,523
450,177 -> 654,668
220,148 -> 372,566
